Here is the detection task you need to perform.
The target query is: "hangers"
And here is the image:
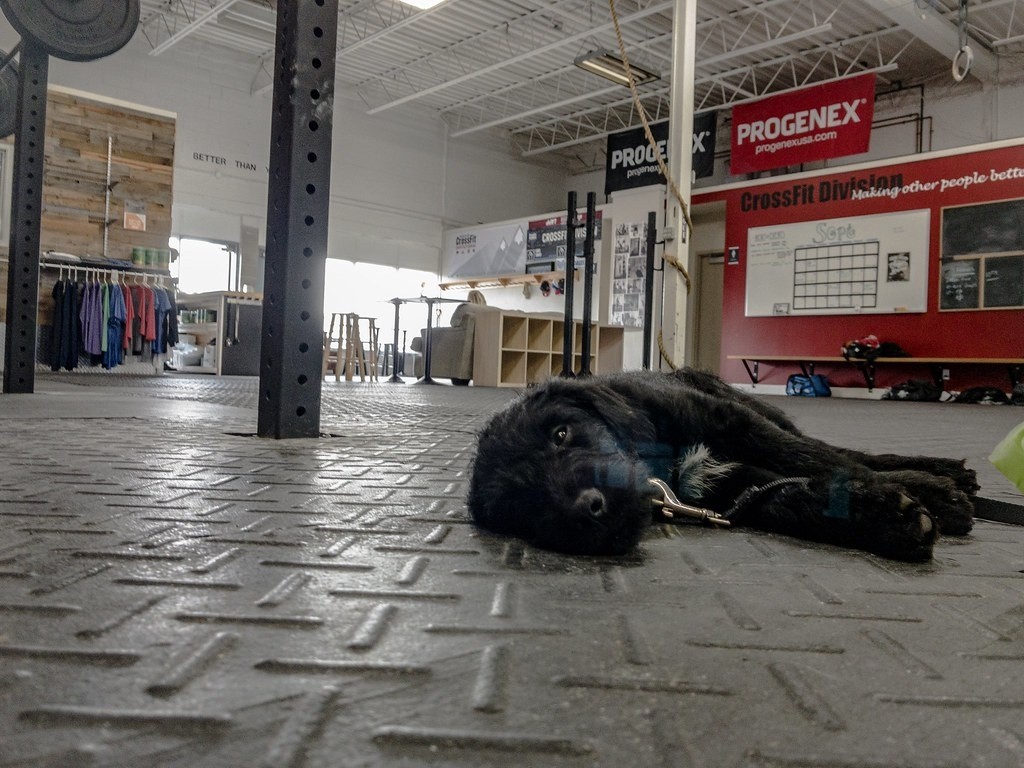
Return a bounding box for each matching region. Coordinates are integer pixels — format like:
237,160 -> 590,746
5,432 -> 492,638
58,263 -> 170,292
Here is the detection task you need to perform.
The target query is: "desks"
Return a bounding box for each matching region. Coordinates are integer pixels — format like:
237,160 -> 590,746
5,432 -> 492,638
163,291 -> 263,376
377,298 -> 471,386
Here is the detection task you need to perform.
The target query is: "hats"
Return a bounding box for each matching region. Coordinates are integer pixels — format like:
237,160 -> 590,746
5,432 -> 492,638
521,282 -> 531,299
551,278 -> 564,295
539,280 -> 550,297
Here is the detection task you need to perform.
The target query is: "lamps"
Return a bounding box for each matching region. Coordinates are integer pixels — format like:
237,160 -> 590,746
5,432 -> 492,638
573,0 -> 662,89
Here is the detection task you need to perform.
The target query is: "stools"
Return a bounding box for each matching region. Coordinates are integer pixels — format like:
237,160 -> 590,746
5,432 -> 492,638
321,312 -> 366,383
346,317 -> 378,383
382,344 -> 394,376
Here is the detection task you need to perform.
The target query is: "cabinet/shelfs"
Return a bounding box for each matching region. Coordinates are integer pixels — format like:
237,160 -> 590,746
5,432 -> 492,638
472,310 -> 625,388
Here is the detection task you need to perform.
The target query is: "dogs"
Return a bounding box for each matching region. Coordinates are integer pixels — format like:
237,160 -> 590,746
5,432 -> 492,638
468,367 -> 980,564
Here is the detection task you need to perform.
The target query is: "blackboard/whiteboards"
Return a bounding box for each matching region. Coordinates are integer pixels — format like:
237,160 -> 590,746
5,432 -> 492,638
743,206 -> 932,318
935,196 -> 1024,311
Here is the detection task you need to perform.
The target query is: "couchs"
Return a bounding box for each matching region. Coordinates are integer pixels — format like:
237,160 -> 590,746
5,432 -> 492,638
410,312 -> 474,387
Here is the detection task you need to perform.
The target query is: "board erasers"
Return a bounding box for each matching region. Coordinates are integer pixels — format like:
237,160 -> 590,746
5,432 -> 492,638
894,305 -> 908,313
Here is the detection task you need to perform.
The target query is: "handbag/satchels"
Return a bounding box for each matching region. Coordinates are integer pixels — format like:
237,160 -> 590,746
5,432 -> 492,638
953,386 -> 1013,406
841,335 -> 881,393
1013,384 -> 1024,406
786,373 -> 832,397
880,379 -> 944,402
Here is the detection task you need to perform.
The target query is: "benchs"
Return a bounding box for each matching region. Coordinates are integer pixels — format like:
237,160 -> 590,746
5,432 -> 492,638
726,354 -> 1023,389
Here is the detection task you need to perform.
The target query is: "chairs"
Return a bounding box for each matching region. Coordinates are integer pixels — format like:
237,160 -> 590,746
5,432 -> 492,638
322,332 -> 370,376
